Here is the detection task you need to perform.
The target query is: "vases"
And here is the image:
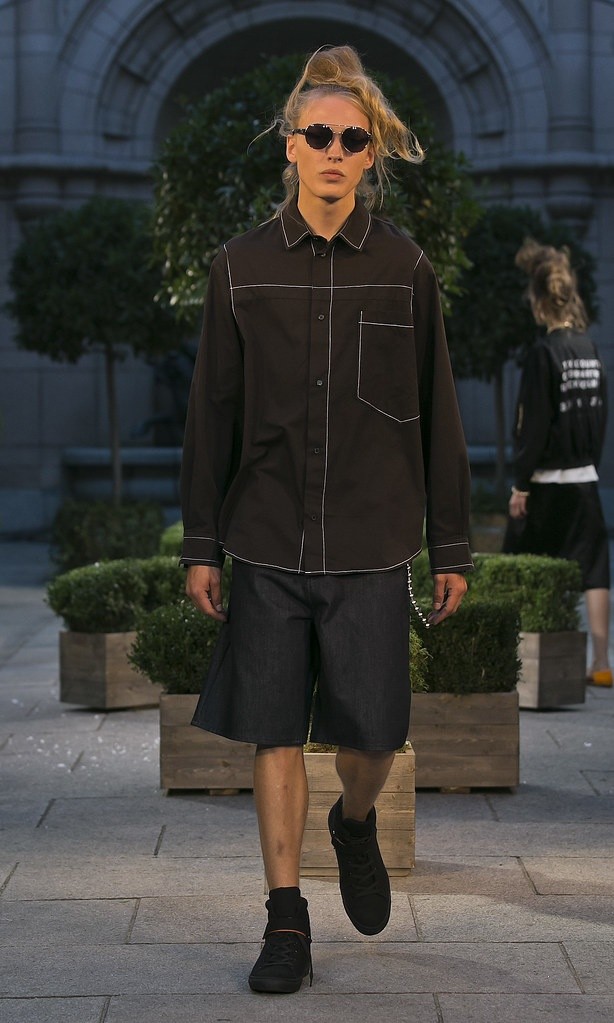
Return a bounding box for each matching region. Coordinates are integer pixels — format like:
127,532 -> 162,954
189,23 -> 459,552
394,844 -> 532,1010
298,734 -> 416,878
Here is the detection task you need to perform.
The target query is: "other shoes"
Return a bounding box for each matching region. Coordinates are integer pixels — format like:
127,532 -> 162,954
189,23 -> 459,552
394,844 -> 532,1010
586,667 -> 614,687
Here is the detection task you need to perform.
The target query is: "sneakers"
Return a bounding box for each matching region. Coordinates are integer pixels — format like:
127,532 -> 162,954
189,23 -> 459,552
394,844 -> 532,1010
329,794 -> 392,935
248,895 -> 312,994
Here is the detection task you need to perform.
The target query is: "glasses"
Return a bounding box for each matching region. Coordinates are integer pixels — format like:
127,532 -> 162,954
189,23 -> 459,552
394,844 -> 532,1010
288,123 -> 374,154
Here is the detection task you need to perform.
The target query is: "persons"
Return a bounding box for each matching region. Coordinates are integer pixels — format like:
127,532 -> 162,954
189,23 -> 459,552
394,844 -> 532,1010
502,238 -> 614,690
178,43 -> 473,995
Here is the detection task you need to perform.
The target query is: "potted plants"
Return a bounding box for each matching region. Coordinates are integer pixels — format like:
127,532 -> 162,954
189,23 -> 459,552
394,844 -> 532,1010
126,597 -> 256,797
41,555 -> 186,709
473,552 -> 588,709
406,603 -> 522,793
470,485 -> 509,552
